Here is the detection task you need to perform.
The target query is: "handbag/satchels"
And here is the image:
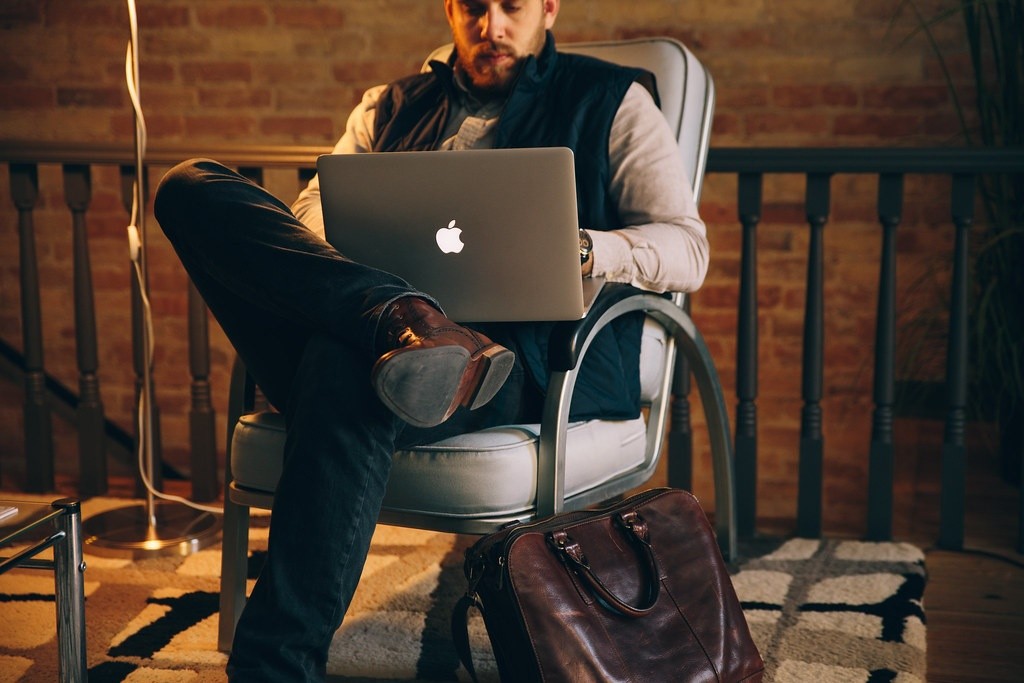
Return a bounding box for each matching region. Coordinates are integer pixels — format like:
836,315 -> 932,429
463,487 -> 766,683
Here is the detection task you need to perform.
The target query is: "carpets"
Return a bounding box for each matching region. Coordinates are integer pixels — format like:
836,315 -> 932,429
0,490 -> 927,680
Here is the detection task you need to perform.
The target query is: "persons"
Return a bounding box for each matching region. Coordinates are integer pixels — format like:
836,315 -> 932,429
153,0 -> 711,683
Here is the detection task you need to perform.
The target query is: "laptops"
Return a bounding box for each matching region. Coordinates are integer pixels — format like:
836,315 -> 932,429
317,146 -> 606,322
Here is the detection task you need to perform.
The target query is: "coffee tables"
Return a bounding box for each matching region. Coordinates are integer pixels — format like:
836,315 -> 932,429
1,499 -> 90,683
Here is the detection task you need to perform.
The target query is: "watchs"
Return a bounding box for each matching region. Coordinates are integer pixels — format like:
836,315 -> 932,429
579,228 -> 593,266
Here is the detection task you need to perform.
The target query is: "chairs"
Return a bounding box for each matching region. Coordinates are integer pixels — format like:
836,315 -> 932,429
210,35 -> 716,647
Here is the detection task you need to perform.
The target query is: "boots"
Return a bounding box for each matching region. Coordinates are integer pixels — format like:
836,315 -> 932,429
370,293 -> 515,429
